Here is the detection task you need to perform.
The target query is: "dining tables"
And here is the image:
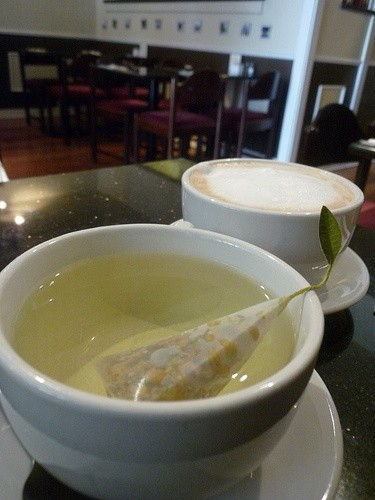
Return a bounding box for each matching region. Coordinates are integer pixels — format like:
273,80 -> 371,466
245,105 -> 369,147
0,158 -> 374,500
98,63 -> 260,161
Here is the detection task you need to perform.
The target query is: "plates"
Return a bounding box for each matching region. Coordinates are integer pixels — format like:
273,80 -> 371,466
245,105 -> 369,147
0,368 -> 344,500
170,217 -> 369,314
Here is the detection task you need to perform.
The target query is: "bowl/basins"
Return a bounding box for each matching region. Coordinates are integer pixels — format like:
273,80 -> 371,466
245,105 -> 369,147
0,218 -> 325,500
181,158 -> 366,292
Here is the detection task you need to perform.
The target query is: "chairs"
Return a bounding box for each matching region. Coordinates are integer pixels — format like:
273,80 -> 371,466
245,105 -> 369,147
309,103 -> 366,163
19,45 -> 287,163
